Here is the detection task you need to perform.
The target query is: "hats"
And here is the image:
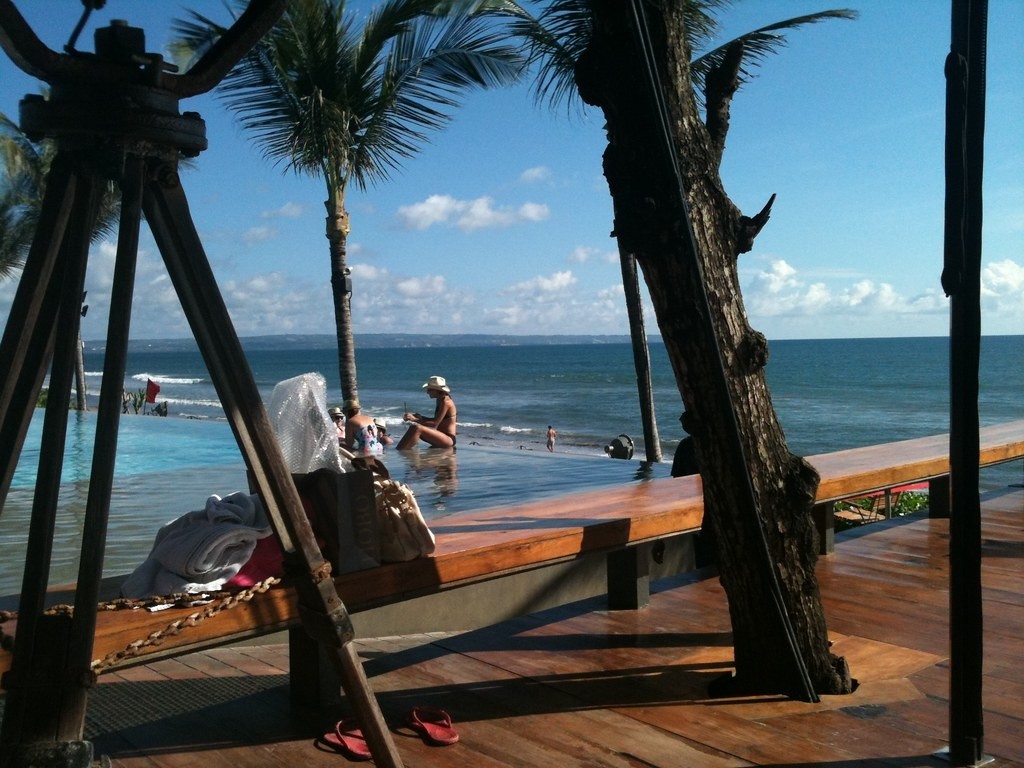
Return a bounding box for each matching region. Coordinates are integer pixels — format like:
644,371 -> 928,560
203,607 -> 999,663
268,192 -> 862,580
421,375 -> 451,393
372,418 -> 387,434
340,400 -> 362,412
327,407 -> 344,417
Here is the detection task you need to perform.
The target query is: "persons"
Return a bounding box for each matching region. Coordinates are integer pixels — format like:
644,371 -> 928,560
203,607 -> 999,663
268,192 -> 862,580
396,376 -> 457,449
544,425 -> 558,452
670,412 -> 702,478
328,400 -> 393,457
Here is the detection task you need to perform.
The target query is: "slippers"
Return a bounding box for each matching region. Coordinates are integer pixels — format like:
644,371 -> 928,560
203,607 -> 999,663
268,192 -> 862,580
316,716 -> 376,762
400,703 -> 460,746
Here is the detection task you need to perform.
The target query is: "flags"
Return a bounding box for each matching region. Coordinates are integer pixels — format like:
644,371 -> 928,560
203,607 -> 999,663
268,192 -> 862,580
146,378 -> 160,403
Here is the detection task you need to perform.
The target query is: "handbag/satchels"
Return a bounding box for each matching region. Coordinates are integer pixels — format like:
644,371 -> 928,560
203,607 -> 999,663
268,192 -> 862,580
339,445 -> 437,564
246,455 -> 384,578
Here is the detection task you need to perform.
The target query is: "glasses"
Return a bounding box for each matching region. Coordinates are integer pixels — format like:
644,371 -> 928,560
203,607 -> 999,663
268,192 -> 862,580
335,418 -> 343,419
343,412 -> 345,414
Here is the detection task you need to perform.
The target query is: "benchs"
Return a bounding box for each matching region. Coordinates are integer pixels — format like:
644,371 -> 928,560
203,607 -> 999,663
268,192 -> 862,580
0,418 -> 1024,699
